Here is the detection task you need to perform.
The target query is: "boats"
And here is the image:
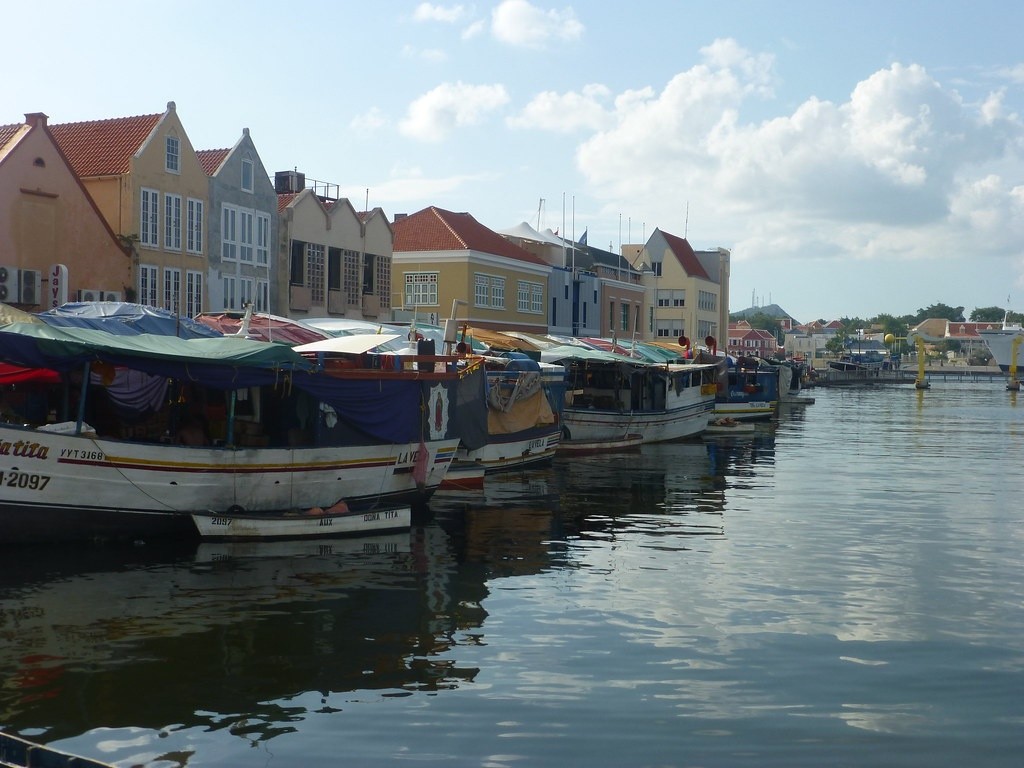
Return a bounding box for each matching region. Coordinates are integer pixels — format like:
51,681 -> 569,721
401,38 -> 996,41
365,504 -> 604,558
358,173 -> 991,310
702,422 -> 755,434
186,528 -> 412,565
176,505 -> 412,542
0,300 -> 801,566
975,294 -> 1024,381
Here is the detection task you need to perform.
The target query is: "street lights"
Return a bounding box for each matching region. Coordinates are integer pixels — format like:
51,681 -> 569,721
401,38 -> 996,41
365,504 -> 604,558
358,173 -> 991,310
856,324 -> 860,354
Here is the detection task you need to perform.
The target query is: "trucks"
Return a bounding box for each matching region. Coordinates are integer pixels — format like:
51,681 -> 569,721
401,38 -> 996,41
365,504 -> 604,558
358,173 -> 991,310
839,355 -> 882,375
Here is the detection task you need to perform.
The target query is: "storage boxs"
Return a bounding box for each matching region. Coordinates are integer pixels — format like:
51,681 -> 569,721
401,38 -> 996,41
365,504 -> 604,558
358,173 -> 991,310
203,404 -> 264,447
